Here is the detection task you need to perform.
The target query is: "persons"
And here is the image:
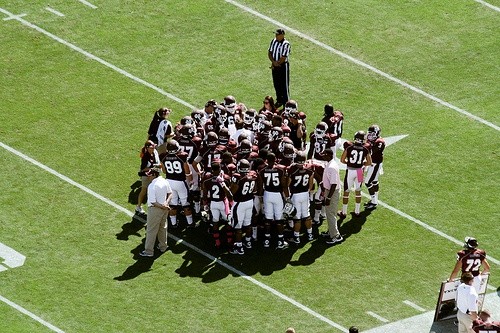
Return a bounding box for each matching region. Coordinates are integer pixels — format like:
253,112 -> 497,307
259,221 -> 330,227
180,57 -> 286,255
268,29 -> 291,108
447,237 -> 500,333
337,125 -> 385,218
135,96 -> 344,257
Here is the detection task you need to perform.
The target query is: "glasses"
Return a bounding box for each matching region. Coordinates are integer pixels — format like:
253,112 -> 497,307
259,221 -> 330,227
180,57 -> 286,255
263,101 -> 270,105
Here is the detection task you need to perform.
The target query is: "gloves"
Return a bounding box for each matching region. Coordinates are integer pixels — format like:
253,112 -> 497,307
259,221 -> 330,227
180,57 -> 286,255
204,205 -> 209,211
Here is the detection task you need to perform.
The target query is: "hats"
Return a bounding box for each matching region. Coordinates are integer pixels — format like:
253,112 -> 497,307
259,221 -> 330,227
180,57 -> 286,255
319,148 -> 333,157
145,168 -> 159,177
273,29 -> 285,35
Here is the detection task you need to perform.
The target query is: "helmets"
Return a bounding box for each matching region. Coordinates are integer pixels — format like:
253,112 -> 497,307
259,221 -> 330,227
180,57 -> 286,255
324,104 -> 333,116
354,131 -> 367,145
367,125 -> 382,141
167,95 -> 328,172
464,236 -> 479,248
283,202 -> 297,219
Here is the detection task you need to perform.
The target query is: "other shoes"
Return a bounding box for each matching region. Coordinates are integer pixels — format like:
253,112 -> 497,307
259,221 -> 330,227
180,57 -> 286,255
172,210 -> 314,255
363,199 -> 378,209
337,211 -> 359,218
311,214 -> 326,225
135,205 -> 145,214
139,250 -> 154,257
157,244 -> 165,252
321,230 -> 343,244
